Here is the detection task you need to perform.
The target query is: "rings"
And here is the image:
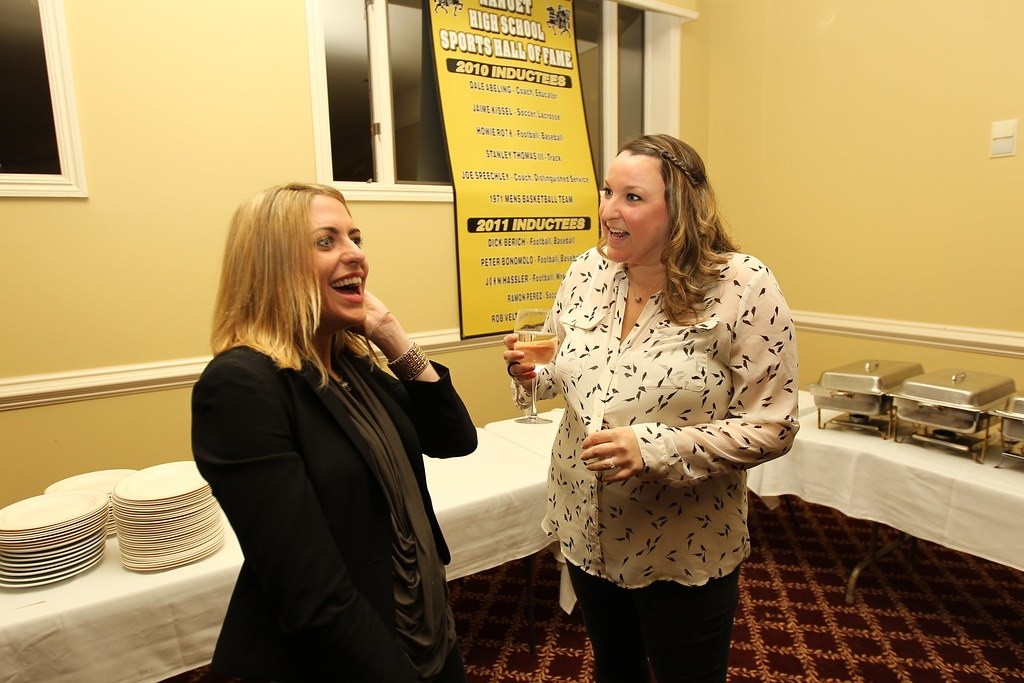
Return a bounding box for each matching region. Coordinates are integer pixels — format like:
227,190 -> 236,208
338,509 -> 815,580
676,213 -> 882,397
609,459 -> 616,469
507,361 -> 520,378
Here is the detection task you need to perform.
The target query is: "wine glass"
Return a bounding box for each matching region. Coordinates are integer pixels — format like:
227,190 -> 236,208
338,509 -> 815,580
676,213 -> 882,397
513,309 -> 556,424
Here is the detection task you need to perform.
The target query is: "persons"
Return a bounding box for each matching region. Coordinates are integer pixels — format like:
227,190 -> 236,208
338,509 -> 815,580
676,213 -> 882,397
190,182 -> 478,683
503,133 -> 800,683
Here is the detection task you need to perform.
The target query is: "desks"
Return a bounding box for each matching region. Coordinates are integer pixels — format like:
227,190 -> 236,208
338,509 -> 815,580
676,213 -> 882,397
0,391 -> 1024,683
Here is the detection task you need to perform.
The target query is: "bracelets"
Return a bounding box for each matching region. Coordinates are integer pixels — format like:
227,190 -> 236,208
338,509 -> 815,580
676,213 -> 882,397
367,310 -> 391,339
385,340 -> 429,380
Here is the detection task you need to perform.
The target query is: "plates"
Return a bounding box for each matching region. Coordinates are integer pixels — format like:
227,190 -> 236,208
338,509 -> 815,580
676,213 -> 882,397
0,461 -> 225,589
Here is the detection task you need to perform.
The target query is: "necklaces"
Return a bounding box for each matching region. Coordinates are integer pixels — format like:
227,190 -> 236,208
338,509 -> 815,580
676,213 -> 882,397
294,342 -> 352,392
625,271 -> 665,303
